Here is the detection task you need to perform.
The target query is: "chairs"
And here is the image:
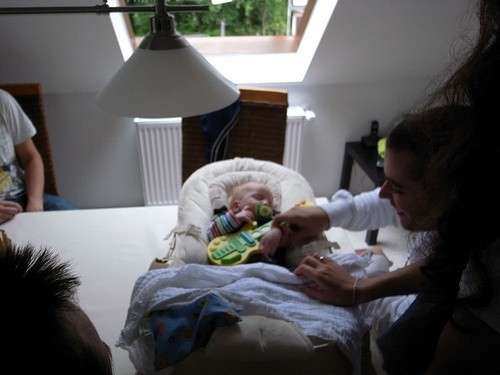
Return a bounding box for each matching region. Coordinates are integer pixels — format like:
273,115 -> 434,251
0,83 -> 60,196
177,85 -> 290,190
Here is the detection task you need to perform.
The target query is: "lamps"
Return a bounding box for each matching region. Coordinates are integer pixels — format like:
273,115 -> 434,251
0,0 -> 243,120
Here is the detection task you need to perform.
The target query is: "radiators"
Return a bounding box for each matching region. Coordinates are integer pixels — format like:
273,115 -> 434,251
135,105 -> 317,206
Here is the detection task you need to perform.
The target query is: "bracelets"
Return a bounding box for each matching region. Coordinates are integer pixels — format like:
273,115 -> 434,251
352,279 -> 360,305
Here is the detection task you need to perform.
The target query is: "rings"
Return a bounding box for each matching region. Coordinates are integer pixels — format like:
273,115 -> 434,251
319,255 -> 325,263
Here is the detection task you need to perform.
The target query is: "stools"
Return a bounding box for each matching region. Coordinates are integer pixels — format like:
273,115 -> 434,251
339,137 -> 398,246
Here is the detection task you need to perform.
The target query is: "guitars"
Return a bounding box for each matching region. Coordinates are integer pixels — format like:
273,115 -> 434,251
208,200 -> 313,266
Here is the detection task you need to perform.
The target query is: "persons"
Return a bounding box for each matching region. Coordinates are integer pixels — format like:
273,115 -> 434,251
273,0 -> 500,375
208,181 -> 297,264
0,229 -> 113,375
0,89 -> 78,224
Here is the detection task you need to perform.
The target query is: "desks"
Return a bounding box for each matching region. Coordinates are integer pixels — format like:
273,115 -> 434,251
0,202 -> 412,375
